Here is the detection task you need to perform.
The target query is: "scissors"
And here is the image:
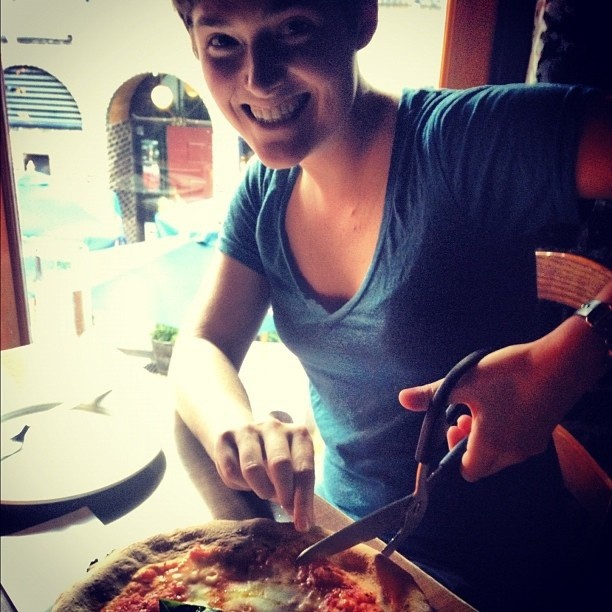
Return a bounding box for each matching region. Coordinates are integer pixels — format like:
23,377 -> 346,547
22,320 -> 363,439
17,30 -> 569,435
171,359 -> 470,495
294,351 -> 485,570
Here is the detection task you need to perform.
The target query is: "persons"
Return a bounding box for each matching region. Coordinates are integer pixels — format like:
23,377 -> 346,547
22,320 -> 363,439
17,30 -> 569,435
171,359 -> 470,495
166,1 -> 612,532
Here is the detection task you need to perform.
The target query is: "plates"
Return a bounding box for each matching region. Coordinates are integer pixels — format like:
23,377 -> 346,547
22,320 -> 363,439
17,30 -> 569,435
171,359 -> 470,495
0,403 -> 170,506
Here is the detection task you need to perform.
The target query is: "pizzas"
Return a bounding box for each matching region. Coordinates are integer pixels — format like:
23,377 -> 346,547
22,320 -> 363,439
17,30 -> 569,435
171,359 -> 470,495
50,518 -> 432,611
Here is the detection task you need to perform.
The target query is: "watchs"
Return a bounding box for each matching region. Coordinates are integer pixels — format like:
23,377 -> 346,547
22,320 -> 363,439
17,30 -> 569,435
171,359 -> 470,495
575,300 -> 612,352
